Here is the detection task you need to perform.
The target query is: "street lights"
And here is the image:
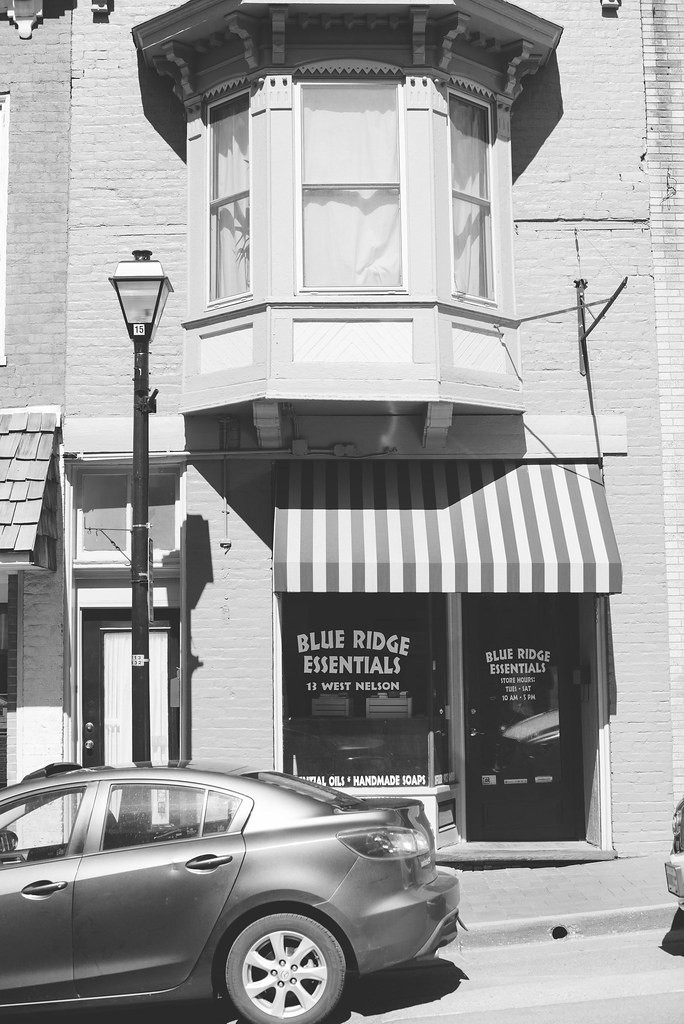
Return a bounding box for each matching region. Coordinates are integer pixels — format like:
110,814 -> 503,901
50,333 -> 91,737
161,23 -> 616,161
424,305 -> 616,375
108,249 -> 176,766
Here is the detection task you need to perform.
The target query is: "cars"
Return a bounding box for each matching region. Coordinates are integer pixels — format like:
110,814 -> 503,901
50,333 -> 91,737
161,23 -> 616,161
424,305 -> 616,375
281,679 -> 589,842
1,762 -> 469,1024
